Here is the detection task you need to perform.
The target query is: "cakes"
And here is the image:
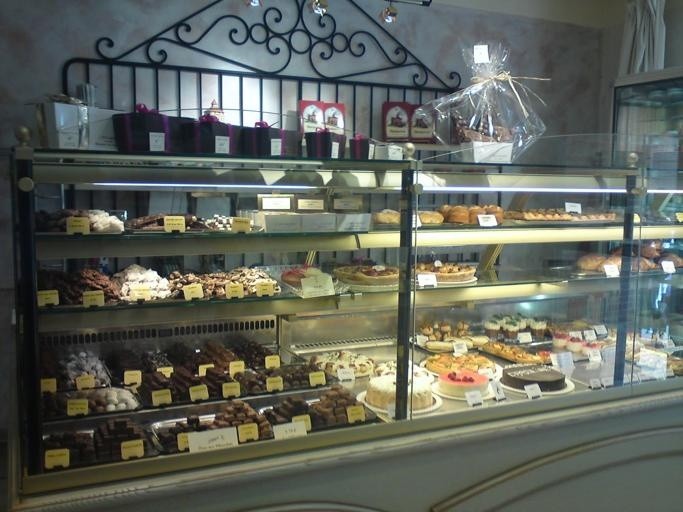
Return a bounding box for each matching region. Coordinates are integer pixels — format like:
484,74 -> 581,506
311,312 -> 599,412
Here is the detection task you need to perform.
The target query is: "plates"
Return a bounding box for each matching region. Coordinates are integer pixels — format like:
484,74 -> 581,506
337,280 -> 399,291
432,380 -> 497,402
411,277 -> 479,285
356,387 -> 443,416
420,356 -> 504,380
497,373 -> 575,394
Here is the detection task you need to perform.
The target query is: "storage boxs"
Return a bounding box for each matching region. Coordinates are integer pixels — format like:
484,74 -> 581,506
308,129 -> 348,162
350,137 -> 377,160
173,114 -> 305,162
374,142 -> 406,163
448,114 -> 519,164
108,113 -> 170,153
34,101 -> 127,157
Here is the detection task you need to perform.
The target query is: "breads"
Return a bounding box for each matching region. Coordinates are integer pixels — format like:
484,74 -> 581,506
507,206 -> 683,273
566,319 -> 683,378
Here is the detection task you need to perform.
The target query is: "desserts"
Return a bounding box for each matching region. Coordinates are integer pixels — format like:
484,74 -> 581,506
37,203 -> 505,473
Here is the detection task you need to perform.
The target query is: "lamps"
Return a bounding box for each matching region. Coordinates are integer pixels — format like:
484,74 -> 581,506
245,0 -> 431,23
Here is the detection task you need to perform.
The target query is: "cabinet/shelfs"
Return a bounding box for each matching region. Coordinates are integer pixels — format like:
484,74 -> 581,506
9,164 -> 683,499
